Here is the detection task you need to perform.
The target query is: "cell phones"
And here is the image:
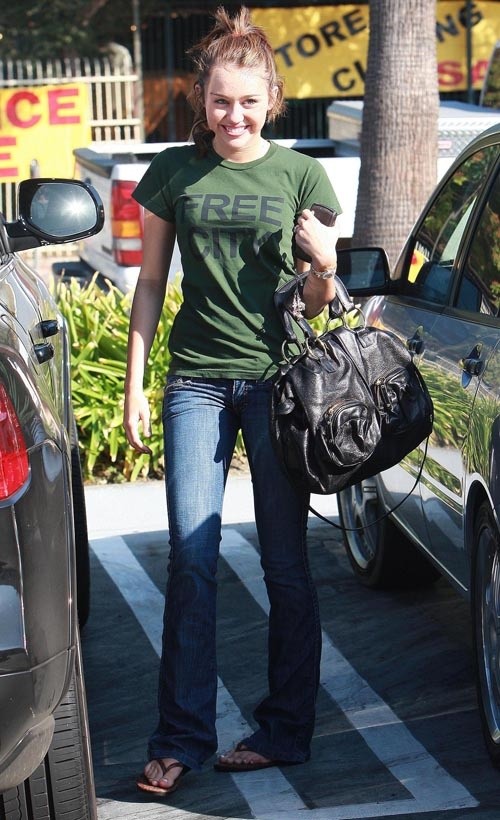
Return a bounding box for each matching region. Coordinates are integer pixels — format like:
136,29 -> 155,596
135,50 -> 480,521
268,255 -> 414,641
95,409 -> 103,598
294,202 -> 338,263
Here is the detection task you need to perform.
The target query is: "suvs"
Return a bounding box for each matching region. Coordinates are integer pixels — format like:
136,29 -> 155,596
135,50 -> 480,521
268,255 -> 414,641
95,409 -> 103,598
1,180 -> 106,820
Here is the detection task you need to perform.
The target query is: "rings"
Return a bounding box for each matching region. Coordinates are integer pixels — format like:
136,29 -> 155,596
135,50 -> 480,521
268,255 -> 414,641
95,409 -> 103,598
293,226 -> 300,233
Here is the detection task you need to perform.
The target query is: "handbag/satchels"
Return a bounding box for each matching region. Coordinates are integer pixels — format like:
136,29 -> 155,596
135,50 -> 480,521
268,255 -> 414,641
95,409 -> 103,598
268,269 -> 434,495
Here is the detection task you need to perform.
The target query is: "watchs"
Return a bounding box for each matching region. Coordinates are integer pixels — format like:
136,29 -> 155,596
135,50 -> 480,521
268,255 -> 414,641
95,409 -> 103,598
311,266 -> 337,281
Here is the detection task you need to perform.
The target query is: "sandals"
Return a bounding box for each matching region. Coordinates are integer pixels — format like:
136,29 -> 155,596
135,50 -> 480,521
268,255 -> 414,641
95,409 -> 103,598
136,757 -> 192,793
213,739 -> 284,770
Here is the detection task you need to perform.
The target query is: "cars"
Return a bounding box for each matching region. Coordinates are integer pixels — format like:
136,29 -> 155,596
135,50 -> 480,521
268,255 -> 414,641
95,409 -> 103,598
331,127 -> 499,762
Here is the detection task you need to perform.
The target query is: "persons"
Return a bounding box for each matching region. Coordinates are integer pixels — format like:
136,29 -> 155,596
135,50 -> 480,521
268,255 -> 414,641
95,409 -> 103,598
123,14 -> 343,796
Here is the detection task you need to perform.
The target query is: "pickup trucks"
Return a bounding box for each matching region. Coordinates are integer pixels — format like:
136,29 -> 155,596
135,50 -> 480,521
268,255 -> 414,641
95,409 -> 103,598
73,103 -> 499,303
